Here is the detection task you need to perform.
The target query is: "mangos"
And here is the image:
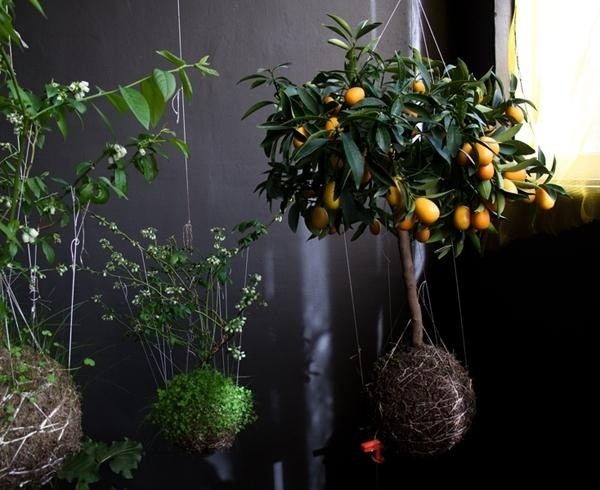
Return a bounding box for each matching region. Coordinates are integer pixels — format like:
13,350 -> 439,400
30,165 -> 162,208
293,80 -> 557,241
